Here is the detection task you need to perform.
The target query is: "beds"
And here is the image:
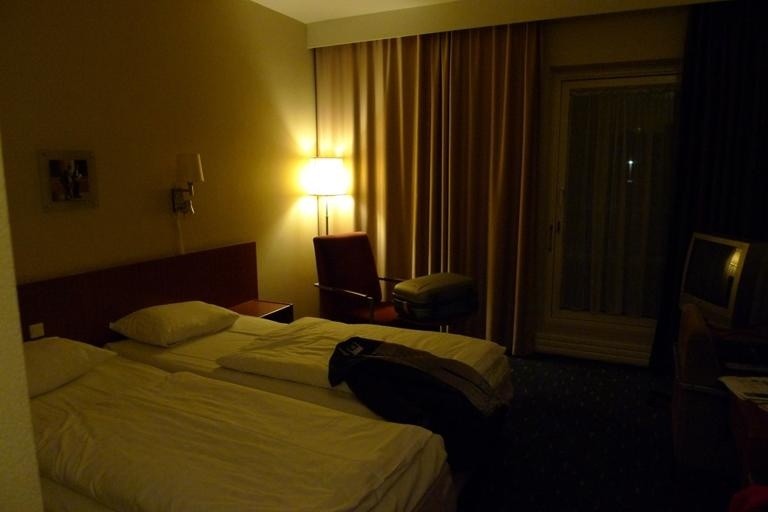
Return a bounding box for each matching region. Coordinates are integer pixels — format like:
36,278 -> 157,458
21,323 -> 451,510
103,298 -> 511,457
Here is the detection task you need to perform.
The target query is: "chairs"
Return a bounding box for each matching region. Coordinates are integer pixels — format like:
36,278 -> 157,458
310,231 -> 406,324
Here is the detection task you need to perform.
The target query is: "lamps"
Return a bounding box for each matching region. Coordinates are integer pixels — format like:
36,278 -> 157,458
171,151 -> 205,216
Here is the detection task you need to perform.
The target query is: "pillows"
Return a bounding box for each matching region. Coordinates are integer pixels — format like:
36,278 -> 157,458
21,334 -> 120,400
109,298 -> 243,349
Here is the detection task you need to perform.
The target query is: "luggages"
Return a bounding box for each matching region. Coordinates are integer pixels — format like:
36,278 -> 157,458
392,273 -> 479,321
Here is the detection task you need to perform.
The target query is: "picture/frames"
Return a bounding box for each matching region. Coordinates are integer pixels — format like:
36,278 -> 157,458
38,149 -> 99,210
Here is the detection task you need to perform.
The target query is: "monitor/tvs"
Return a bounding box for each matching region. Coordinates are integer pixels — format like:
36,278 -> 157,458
679,232 -> 768,332
